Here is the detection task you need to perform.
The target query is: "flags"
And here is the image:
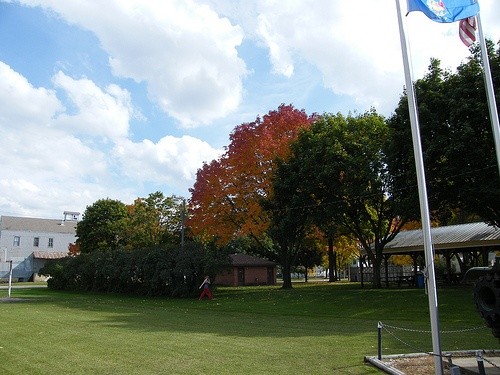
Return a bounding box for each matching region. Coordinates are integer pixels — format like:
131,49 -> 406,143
404,0 -> 479,23
459,15 -> 481,47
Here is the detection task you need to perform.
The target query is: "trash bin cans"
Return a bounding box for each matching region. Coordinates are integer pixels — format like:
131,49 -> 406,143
416,272 -> 425,289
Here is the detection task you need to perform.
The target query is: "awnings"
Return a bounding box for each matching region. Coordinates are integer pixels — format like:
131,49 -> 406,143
32,251 -> 74,259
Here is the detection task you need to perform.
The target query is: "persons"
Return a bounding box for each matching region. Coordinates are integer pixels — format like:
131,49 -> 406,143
198,275 -> 215,302
